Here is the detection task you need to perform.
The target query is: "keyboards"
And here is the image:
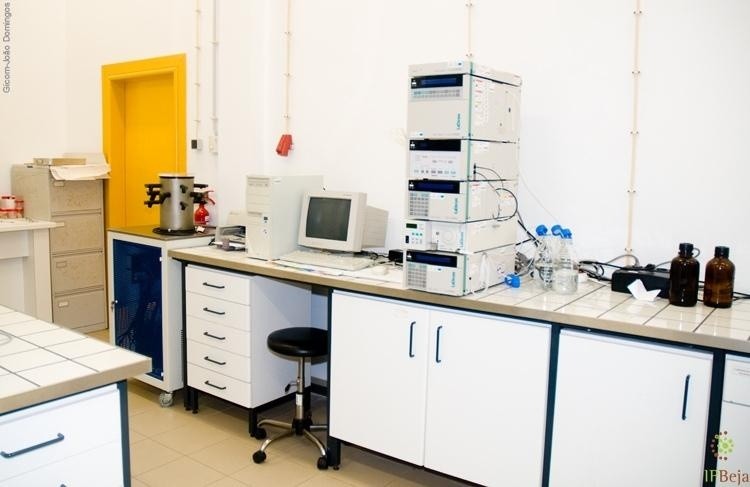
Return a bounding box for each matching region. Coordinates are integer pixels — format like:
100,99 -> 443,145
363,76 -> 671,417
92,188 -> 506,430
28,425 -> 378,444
272,250 -> 374,272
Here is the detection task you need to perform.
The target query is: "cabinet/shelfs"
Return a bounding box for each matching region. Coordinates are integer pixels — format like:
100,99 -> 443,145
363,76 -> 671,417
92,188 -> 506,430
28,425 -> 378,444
0,385 -> 125,487
181,261 -> 251,440
328,290 -> 552,486
714,353 -> 750,487
550,328 -> 715,487
106,229 -> 169,393
47,166 -> 107,337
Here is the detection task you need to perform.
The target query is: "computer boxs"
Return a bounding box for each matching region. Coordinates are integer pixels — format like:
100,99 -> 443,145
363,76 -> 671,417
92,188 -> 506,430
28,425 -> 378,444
245,173 -> 323,261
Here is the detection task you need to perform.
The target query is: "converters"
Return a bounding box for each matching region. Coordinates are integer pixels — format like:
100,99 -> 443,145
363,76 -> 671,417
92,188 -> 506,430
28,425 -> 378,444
611,266 -> 670,298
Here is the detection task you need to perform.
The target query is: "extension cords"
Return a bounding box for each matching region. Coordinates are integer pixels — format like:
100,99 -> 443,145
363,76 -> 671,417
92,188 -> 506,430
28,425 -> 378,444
577,263 -> 588,284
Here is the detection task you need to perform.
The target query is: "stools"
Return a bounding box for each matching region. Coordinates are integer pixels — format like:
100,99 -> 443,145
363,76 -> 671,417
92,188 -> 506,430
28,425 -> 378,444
253,326 -> 329,470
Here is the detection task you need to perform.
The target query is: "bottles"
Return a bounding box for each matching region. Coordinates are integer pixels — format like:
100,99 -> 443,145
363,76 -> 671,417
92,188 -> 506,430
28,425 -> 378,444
8,209 -> 16,219
17,210 -> 23,219
703,246 -> 735,309
533,225 -> 554,290
552,225 -> 578,294
668,242 -> 700,307
0,208 -> 8,219
15,200 -> 24,210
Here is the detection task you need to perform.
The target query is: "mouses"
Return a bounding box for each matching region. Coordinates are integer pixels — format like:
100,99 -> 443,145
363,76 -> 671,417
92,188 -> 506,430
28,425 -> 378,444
373,264 -> 389,276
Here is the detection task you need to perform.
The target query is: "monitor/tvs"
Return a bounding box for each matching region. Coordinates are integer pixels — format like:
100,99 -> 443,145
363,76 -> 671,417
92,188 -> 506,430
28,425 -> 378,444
298,191 -> 389,253
